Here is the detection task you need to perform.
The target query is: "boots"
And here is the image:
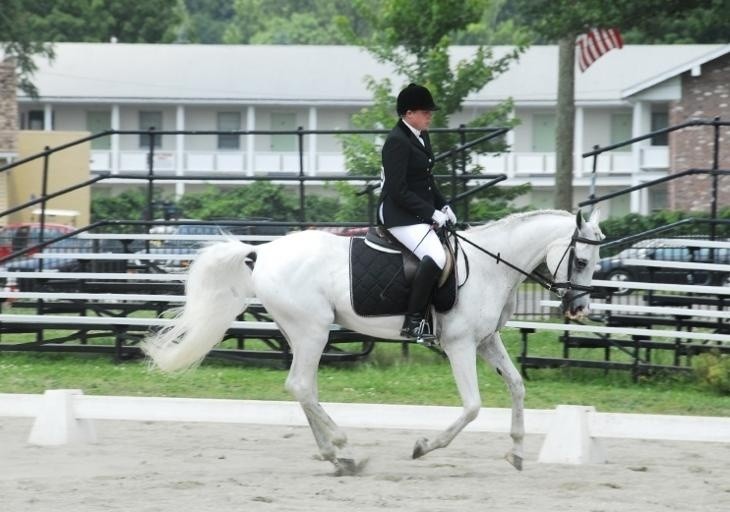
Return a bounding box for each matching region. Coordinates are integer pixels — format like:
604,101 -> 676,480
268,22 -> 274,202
400,256 -> 442,340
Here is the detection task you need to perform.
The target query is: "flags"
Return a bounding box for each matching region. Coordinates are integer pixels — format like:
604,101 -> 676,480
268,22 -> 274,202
574,23 -> 624,72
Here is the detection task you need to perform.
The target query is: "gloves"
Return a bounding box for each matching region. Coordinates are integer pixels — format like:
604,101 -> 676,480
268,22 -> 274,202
432,205 -> 456,228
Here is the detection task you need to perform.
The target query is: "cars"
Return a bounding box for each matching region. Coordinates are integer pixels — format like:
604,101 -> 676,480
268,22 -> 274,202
0,218 -> 293,306
591,237 -> 730,295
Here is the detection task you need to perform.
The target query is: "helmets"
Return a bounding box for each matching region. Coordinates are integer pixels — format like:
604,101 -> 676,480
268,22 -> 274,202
397,83 -> 441,116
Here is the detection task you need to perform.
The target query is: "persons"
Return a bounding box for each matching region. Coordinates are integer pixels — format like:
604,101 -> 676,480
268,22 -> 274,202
376,83 -> 458,341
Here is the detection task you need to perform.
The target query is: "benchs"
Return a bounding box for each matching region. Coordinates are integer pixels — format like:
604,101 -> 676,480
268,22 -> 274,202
501,239 -> 730,383
1,237 -> 381,364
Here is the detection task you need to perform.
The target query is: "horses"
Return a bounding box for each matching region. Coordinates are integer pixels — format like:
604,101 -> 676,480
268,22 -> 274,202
137,205 -> 608,471
